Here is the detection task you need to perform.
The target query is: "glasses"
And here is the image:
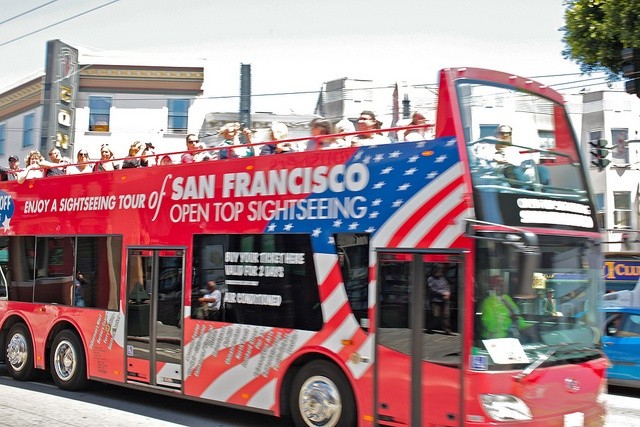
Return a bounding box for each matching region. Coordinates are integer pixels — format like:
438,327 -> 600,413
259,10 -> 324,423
497,132 -> 511,135
358,118 -> 372,121
101,144 -> 109,147
188,140 -> 199,144
79,154 -> 87,156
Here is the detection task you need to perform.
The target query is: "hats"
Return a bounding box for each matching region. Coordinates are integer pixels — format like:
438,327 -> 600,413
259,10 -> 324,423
412,113 -> 428,122
545,288 -> 555,293
361,110 -> 383,126
8,155 -> 19,161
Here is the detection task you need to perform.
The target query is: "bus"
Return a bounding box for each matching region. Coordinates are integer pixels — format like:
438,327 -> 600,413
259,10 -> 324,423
0,65 -> 612,427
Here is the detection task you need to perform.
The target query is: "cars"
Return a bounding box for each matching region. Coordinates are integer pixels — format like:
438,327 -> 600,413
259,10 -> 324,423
572,304 -> 640,389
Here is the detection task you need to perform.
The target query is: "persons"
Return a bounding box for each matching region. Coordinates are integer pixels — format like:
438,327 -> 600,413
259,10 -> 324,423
181,133 -> 212,163
140,142 -> 155,167
69,268 -> 89,306
216,120 -> 255,159
92,142 -> 119,173
492,123 -> 522,165
197,280 -> 222,320
160,154 -> 174,165
350,108 -> 396,146
427,262 -> 452,335
330,118 -> 357,148
122,140 -> 142,168
541,288 -> 558,316
304,117 -> 339,150
404,110 -> 429,141
17,149 -> 47,185
479,275 -> 533,339
45,147 -> 71,178
259,122 -> 300,157
1,153 -> 27,181
65,149 -> 92,175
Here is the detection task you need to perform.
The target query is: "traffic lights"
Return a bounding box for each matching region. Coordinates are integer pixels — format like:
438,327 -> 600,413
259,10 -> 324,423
597,137 -> 609,167
588,139 -> 598,167
